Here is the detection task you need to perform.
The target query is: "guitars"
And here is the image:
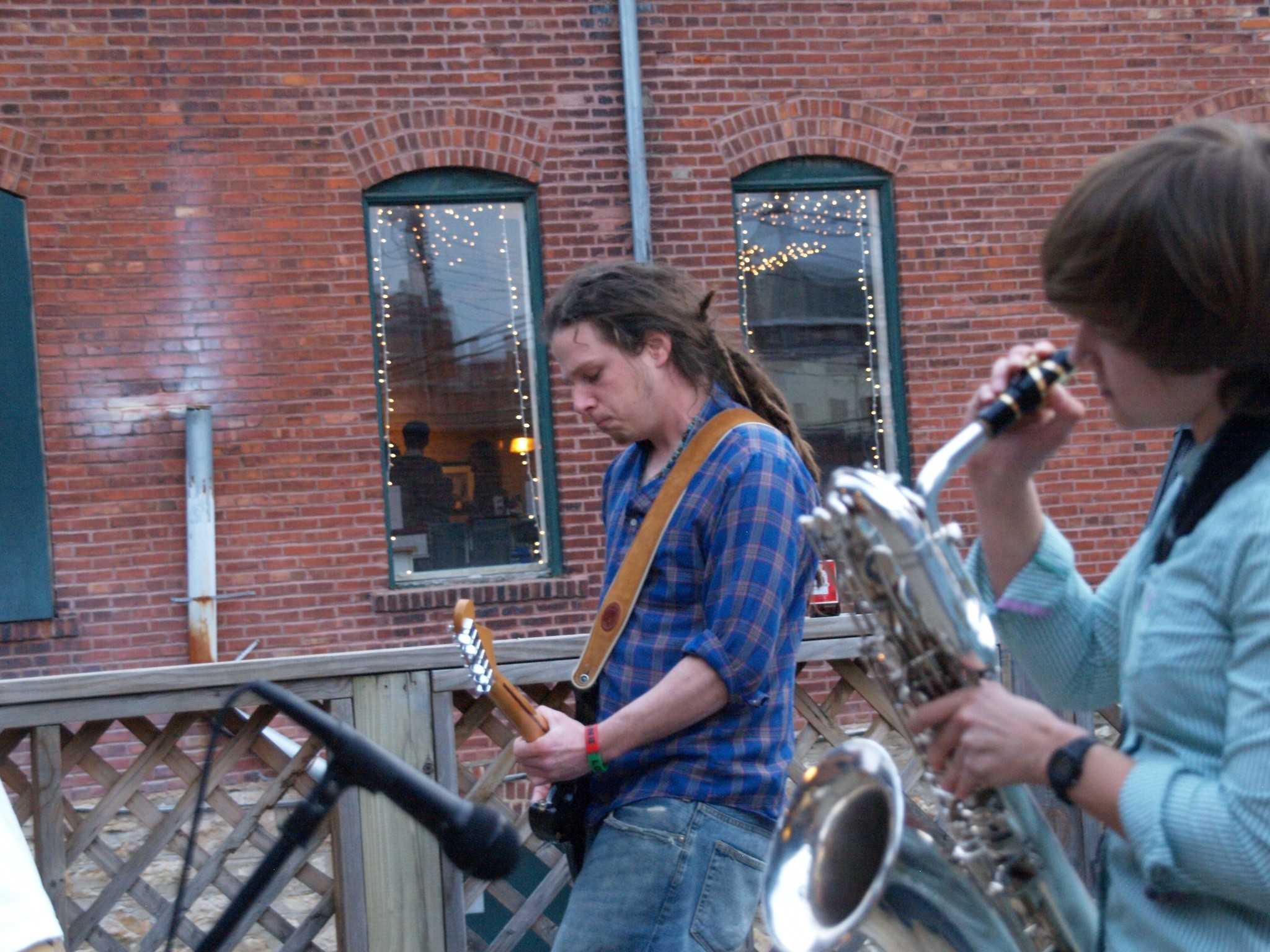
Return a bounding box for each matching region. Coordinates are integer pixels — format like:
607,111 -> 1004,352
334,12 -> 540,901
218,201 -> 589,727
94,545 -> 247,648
447,598 -> 591,886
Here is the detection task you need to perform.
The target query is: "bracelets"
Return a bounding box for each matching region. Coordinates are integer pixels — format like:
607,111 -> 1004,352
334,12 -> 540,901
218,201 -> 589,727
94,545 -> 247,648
585,724 -> 609,775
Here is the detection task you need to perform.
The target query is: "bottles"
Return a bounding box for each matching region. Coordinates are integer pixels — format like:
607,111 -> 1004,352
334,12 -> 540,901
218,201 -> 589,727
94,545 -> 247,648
806,555 -> 840,616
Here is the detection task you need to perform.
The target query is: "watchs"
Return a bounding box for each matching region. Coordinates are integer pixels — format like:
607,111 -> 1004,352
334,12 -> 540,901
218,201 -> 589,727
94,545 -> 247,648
1047,736 -> 1100,806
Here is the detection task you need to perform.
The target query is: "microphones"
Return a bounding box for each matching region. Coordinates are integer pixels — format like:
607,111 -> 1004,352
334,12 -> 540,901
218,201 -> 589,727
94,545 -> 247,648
247,677 -> 522,881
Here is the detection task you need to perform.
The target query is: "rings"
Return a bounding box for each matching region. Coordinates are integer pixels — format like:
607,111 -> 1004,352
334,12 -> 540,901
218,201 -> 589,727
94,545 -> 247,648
945,754 -> 960,771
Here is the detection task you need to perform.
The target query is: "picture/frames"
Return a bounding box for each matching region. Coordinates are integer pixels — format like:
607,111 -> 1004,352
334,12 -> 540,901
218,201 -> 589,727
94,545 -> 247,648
438,462 -> 476,515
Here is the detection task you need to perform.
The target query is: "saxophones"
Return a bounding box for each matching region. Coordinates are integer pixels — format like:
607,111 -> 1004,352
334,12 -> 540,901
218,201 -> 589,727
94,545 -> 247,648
764,335 -> 1098,952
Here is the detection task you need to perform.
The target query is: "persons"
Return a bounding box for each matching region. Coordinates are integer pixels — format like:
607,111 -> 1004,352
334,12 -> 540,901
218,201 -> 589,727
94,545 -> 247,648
908,120 -> 1270,952
511,261 -> 824,952
389,420 -> 539,571
